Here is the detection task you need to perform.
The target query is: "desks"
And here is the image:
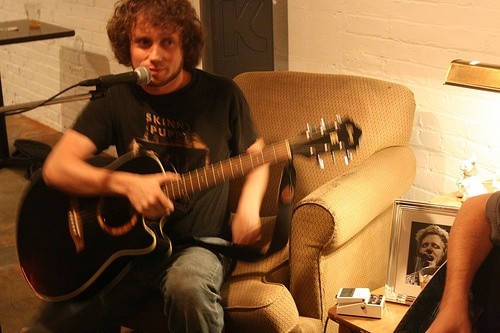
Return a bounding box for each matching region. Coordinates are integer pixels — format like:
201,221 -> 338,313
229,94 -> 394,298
323,285 -> 409,332
0,19 -> 76,166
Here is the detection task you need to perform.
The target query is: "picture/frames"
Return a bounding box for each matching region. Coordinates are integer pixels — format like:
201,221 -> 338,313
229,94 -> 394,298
383,200 -> 460,306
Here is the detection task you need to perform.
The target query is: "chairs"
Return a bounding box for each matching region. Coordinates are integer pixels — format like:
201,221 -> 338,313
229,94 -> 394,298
415,191 -> 500,332
219,70 -> 417,333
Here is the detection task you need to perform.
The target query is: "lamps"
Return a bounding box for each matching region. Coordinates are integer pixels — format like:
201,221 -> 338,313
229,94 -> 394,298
443,59 -> 500,201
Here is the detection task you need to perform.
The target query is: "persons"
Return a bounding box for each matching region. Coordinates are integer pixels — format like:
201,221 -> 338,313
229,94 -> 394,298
31,0 -> 271,333
407,225 -> 449,286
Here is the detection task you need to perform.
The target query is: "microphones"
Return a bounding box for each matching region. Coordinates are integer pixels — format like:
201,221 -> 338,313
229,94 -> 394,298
420,252 -> 431,266
78,66 -> 153,87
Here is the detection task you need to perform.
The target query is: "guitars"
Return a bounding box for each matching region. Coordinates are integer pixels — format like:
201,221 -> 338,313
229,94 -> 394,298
15,113 -> 362,306
